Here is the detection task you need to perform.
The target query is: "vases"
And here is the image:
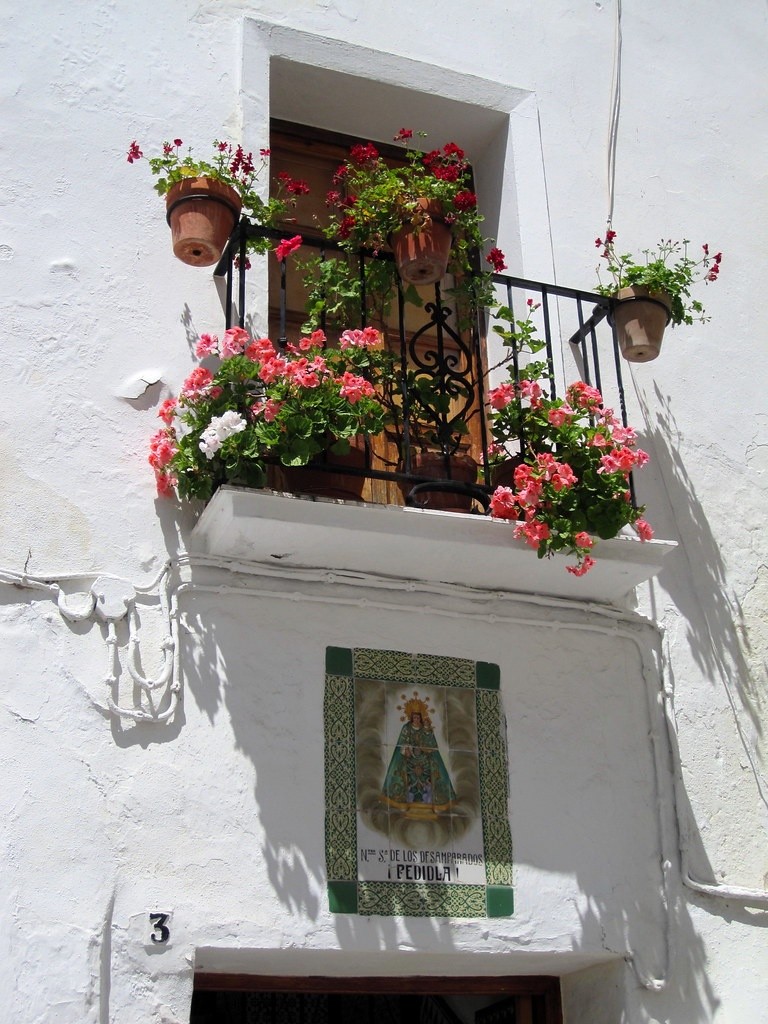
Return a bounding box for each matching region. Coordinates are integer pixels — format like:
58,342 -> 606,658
395,453 -> 477,514
166,177 -> 243,267
286,435 -> 373,495
396,191 -> 444,220
607,286 -> 673,363
387,212 -> 456,286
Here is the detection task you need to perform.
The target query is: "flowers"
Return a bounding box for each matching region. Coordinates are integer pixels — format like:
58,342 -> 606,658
150,324 -> 390,502
593,229 -> 722,324
298,127 -> 547,452
477,358 -> 655,578
126,138 -> 310,269
